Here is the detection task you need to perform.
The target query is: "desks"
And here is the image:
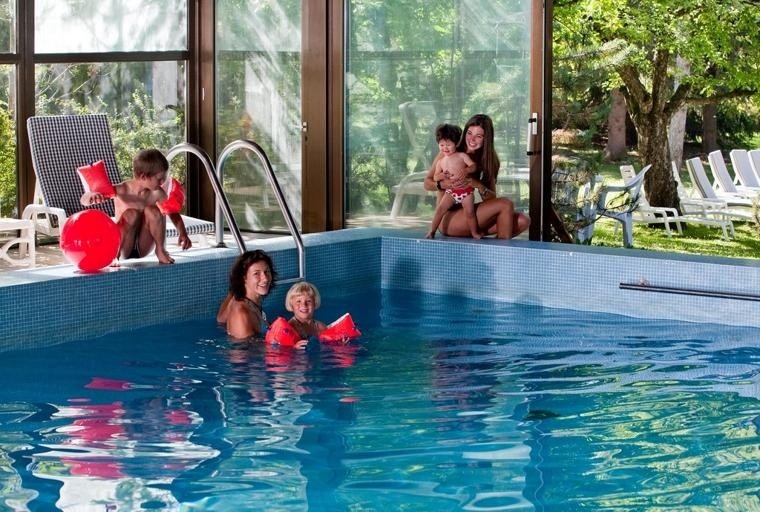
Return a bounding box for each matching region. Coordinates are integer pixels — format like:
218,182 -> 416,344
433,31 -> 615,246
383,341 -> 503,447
0,216 -> 36,269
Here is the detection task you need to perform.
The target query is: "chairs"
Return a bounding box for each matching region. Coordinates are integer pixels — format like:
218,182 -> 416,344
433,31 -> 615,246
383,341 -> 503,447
729,149 -> 760,188
389,99 -> 530,220
551,165 -> 603,245
670,160 -> 752,221
619,165 -> 738,241
707,149 -> 760,198
588,163 -> 652,248
18,114 -> 216,259
685,157 -> 754,210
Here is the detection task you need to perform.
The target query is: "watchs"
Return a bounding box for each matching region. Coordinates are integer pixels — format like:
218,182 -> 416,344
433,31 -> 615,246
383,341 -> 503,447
436,180 -> 442,191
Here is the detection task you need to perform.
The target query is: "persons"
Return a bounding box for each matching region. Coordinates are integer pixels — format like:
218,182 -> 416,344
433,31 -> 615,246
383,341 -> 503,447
424,114 -> 530,241
216,249 -> 278,340
284,281 -> 350,350
425,123 -> 484,241
80,149 -> 193,268
